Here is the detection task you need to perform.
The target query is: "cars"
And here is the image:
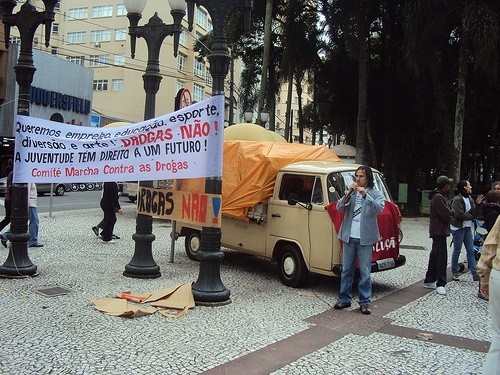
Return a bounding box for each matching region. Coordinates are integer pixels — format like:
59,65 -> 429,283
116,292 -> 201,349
0,176 -> 72,196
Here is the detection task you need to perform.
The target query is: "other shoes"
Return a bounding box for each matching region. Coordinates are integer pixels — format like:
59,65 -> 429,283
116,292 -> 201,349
424,282 -> 437,289
473,273 -> 480,281
0,234 -> 7,248
436,286 -> 446,294
360,305 -> 371,315
452,274 -> 460,281
29,242 -> 44,247
334,302 -> 351,310
100,239 -> 116,244
92,226 -> 99,236
112,234 -> 120,239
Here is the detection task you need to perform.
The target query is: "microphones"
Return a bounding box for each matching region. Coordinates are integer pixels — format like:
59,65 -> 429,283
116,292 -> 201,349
345,188 -> 354,200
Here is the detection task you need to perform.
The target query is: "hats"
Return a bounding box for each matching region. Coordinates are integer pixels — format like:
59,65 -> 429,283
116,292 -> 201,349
437,175 -> 453,186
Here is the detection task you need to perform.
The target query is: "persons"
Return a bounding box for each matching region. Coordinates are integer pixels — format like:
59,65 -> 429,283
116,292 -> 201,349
0,171 -> 44,248
424,176 -> 455,294
476,215 -> 500,375
450,180 -> 500,301
334,166 -> 385,314
93,182 -> 121,244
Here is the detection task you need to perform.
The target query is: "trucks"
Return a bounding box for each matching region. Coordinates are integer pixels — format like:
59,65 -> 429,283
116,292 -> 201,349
171,159 -> 407,290
123,182 -> 139,203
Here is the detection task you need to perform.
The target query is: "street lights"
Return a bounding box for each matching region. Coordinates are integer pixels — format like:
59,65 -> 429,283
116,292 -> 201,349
123,0 -> 188,281
